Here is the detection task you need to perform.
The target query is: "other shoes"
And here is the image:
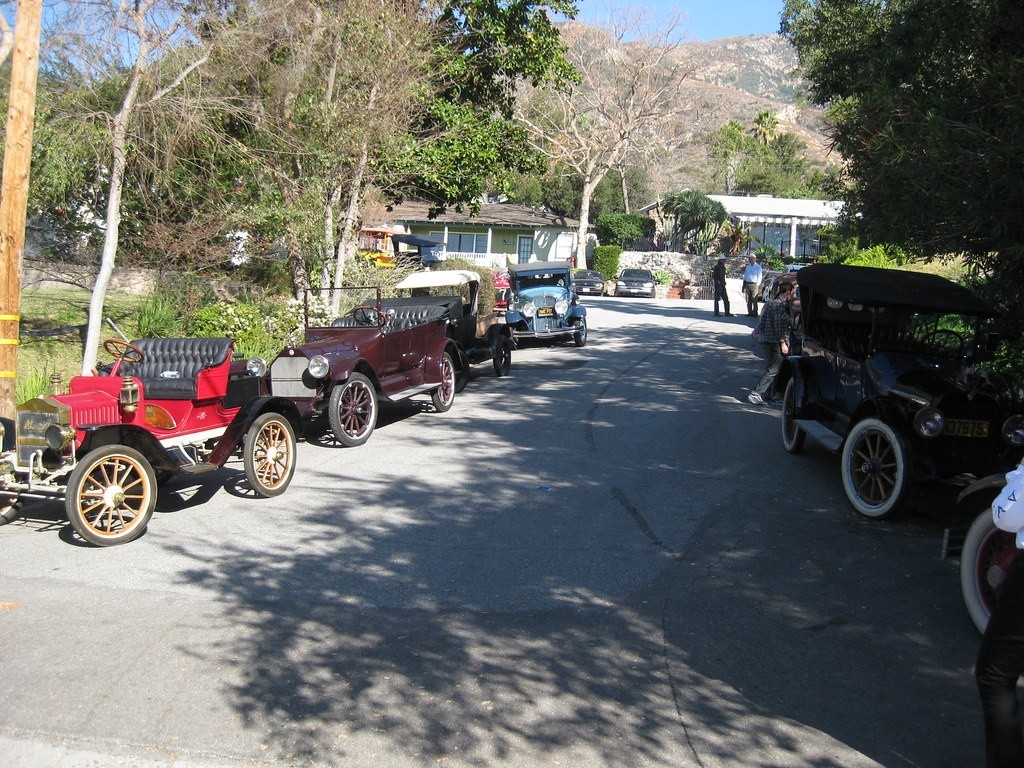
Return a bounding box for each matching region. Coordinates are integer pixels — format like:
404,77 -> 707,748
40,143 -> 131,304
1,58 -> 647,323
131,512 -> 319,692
748,390 -> 768,404
725,312 -> 734,317
747,313 -> 758,317
715,313 -> 723,317
764,395 -> 784,406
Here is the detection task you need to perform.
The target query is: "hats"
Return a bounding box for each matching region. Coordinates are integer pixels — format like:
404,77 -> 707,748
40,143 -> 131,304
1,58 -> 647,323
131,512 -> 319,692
749,254 -> 756,259
719,255 -> 727,259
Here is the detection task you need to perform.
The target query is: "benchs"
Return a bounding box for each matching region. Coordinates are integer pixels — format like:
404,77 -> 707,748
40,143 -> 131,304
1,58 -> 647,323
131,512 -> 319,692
98,337 -> 236,401
809,319 -> 940,362
331,305 -> 449,333
518,277 -> 565,290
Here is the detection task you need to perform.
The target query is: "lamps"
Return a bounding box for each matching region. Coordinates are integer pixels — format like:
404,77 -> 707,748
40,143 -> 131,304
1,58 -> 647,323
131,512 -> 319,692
502,239 -> 514,246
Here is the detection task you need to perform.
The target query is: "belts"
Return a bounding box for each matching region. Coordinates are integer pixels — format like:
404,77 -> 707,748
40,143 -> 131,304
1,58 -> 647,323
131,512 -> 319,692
746,282 -> 756,284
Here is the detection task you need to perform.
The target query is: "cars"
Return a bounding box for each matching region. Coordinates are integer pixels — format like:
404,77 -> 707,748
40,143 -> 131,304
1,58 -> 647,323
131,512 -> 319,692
574,270 -> 608,297
229,287 -> 464,447
394,270 -> 512,393
490,262 -> 587,350
756,261 -> 1024,637
613,269 -> 659,298
0,337 -> 305,547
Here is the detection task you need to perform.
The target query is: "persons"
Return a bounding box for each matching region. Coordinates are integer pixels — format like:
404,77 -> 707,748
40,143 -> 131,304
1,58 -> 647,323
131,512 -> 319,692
713,254 -> 734,317
742,253 -> 762,317
975,456 -> 1024,768
747,282 -> 794,403
